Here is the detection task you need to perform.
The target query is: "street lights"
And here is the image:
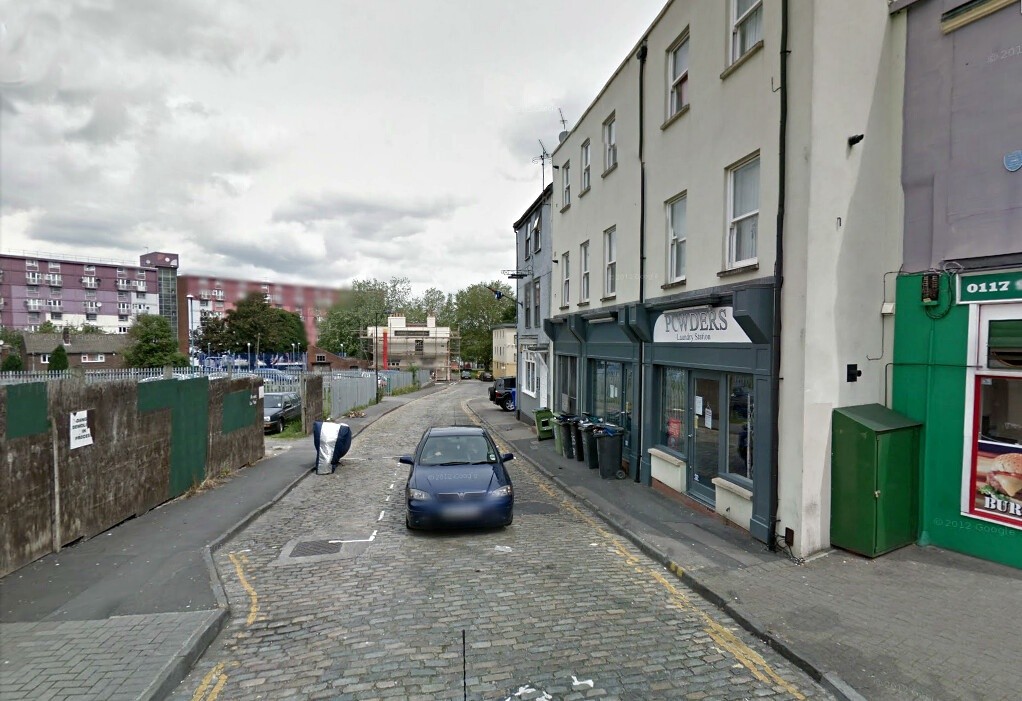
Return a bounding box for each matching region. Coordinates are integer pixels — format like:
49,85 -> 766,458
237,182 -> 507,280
340,343 -> 343,359
247,343 -> 252,374
375,311 -> 395,404
290,343 -> 301,363
186,294 -> 195,375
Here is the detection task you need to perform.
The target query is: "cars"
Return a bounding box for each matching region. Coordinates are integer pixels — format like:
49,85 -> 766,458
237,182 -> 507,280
264,392 -> 301,437
489,377 -> 515,412
140,372 -> 227,382
479,372 -> 493,381
476,371 -> 485,378
461,371 -> 471,379
399,425 -> 515,533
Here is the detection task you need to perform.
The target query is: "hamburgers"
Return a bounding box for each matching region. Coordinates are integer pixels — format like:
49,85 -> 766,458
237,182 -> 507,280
981,453 -> 1022,501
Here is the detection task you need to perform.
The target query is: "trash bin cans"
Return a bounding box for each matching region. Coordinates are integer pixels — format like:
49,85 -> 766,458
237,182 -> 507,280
550,410 -> 591,464
593,424 -> 627,479
579,422 -> 601,470
532,408 -> 554,441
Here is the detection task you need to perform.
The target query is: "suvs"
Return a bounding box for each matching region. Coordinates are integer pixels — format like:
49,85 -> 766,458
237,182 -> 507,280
254,368 -> 299,382
